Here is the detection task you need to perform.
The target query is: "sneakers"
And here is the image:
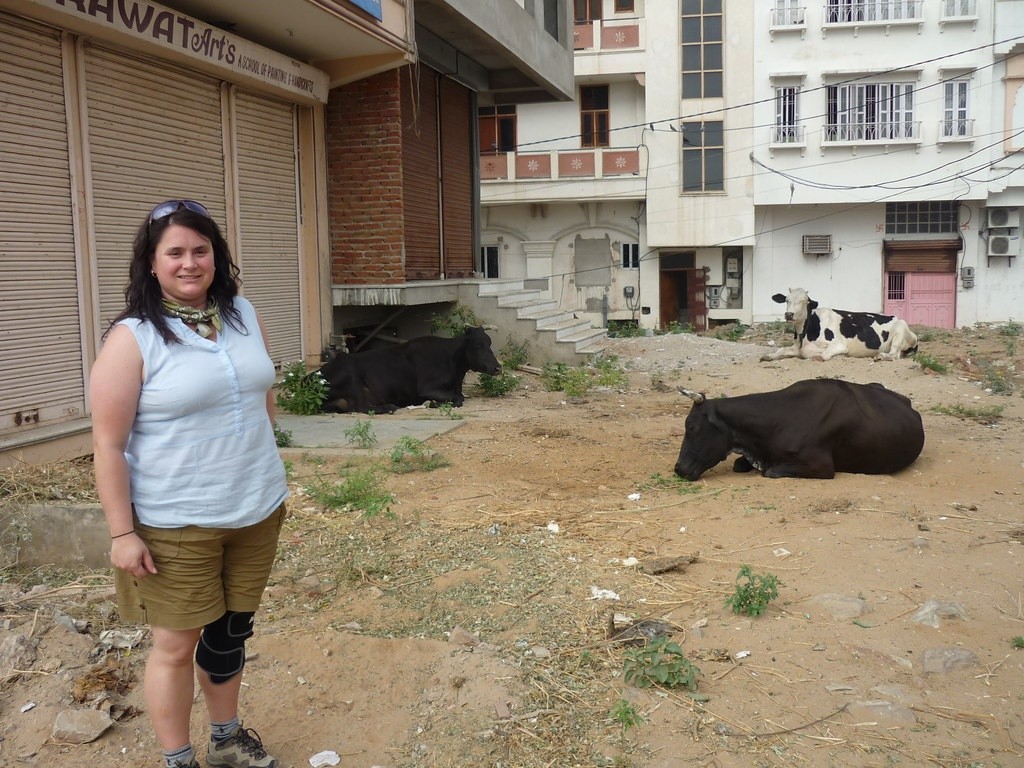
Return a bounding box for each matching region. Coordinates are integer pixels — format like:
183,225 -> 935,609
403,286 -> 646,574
206,720 -> 279,768
172,753 -> 202,768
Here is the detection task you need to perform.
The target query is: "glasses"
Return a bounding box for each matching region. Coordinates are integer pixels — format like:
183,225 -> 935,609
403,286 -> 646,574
149,200 -> 210,233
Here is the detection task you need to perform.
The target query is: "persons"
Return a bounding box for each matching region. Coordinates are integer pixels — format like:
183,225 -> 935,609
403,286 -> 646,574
89,198 -> 293,768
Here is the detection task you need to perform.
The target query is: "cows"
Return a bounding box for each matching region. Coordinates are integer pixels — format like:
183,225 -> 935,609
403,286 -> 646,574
674,378 -> 924,482
301,323 -> 502,416
760,287 -> 919,363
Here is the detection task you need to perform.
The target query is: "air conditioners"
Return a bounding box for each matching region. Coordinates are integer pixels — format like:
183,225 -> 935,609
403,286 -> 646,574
803,234 -> 832,254
987,208 -> 1020,228
987,236 -> 1020,256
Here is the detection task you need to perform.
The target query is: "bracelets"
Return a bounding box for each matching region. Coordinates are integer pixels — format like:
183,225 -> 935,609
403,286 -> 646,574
112,530 -> 135,539
